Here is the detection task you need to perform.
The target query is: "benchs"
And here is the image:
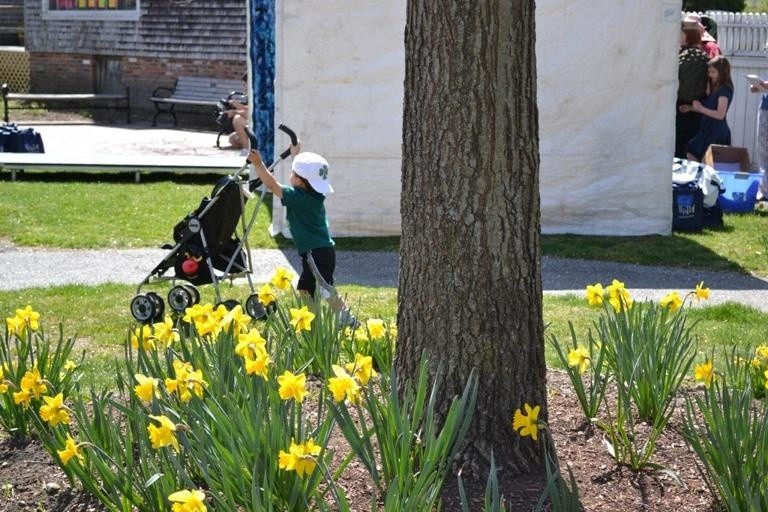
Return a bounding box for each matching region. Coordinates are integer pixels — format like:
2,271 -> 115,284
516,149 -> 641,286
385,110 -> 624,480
148,74 -> 248,126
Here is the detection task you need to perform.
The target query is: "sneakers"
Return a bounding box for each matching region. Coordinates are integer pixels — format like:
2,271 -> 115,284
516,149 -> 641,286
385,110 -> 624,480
338,316 -> 360,331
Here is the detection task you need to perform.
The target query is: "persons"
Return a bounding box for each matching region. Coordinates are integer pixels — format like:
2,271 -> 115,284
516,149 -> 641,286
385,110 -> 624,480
675,14 -> 734,166
248,138 -> 360,330
750,81 -> 768,201
216,71 -> 249,156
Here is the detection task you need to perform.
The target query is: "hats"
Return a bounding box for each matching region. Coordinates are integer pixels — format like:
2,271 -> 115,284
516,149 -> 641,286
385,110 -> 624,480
292,152 -> 335,194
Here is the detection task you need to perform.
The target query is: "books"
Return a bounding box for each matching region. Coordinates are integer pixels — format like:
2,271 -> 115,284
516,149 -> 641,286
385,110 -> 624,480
746,74 -> 768,91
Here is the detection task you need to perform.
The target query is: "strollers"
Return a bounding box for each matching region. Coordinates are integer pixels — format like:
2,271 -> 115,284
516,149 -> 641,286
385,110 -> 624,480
129,124 -> 297,323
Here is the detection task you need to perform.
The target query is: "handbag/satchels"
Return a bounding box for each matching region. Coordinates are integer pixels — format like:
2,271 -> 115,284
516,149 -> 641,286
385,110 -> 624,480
672,157 -> 726,230
5,128 -> 43,153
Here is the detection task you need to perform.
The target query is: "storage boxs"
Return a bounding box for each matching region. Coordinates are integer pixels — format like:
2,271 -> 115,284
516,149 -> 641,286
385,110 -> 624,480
702,143 -> 752,172
715,169 -> 766,217
